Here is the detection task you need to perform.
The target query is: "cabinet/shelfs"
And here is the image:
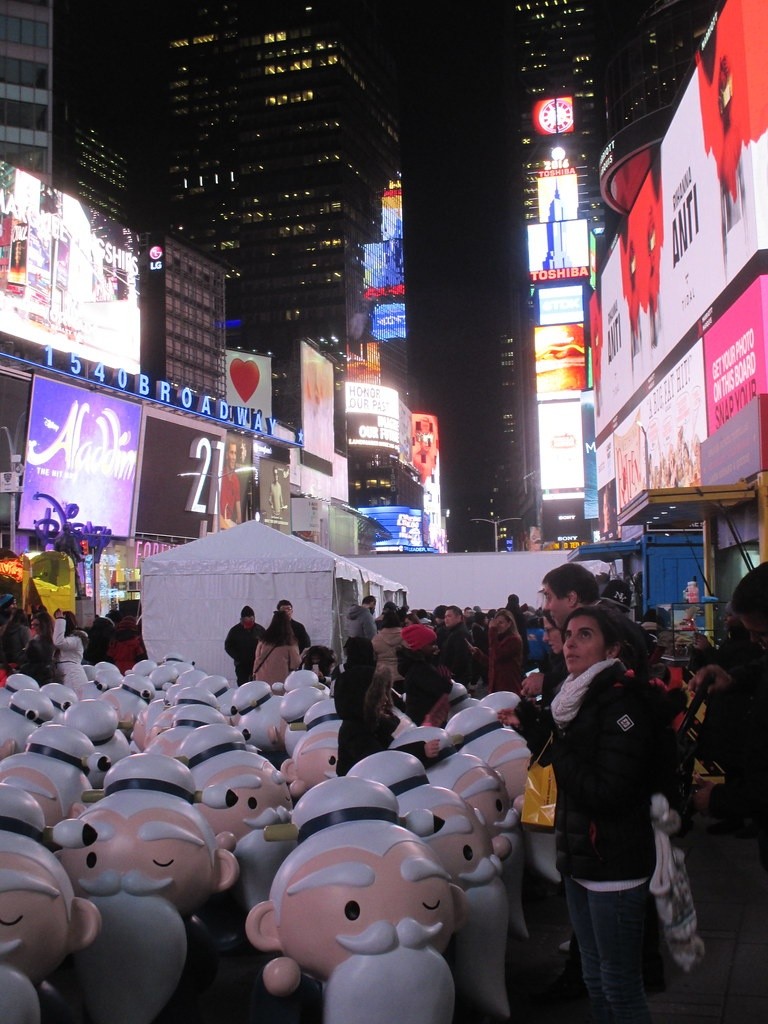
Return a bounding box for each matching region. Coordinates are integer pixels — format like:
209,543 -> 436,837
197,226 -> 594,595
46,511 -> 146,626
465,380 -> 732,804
655,600 -> 728,668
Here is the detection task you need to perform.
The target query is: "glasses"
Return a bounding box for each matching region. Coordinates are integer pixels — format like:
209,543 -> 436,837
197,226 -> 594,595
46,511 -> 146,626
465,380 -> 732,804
543,626 -> 556,636
279,606 -> 292,611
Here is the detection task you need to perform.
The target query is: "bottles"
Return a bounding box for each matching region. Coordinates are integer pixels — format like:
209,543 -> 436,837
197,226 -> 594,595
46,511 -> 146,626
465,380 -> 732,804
687,581 -> 699,604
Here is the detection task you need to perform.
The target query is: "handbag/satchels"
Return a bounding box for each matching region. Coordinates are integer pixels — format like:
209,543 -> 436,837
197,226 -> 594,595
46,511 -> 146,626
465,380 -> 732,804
519,733 -> 559,834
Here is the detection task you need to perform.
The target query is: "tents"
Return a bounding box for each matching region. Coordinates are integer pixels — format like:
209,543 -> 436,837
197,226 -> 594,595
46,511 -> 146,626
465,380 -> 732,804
141,520 -> 409,688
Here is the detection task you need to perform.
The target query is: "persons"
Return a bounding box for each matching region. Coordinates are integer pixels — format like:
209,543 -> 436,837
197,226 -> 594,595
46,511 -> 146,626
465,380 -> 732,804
224,564 -> 768,1024
0,657 -> 537,1024
268,467 -> 288,530
0,594 -> 148,689
650,426 -> 702,486
219,439 -> 243,529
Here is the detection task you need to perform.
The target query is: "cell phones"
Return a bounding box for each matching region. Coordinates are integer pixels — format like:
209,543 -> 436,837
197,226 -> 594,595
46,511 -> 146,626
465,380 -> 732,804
464,638 -> 477,652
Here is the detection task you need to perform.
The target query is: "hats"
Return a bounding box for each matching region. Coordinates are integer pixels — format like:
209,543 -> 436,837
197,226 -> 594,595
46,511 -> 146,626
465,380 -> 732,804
600,580 -> 632,610
648,795 -> 704,970
383,601 -> 399,611
0,594 -> 14,611
400,626 -> 438,652
119,616 -> 137,629
241,606 -> 254,617
433,605 -> 448,619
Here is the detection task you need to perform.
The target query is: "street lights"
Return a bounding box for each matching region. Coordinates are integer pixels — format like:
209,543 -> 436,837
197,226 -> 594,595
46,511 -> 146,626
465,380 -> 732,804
470,517 -> 522,552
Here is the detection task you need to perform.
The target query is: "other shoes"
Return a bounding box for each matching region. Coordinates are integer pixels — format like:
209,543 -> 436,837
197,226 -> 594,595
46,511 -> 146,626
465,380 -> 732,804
738,820 -> 761,839
528,969 -> 590,1003
706,815 -> 745,837
